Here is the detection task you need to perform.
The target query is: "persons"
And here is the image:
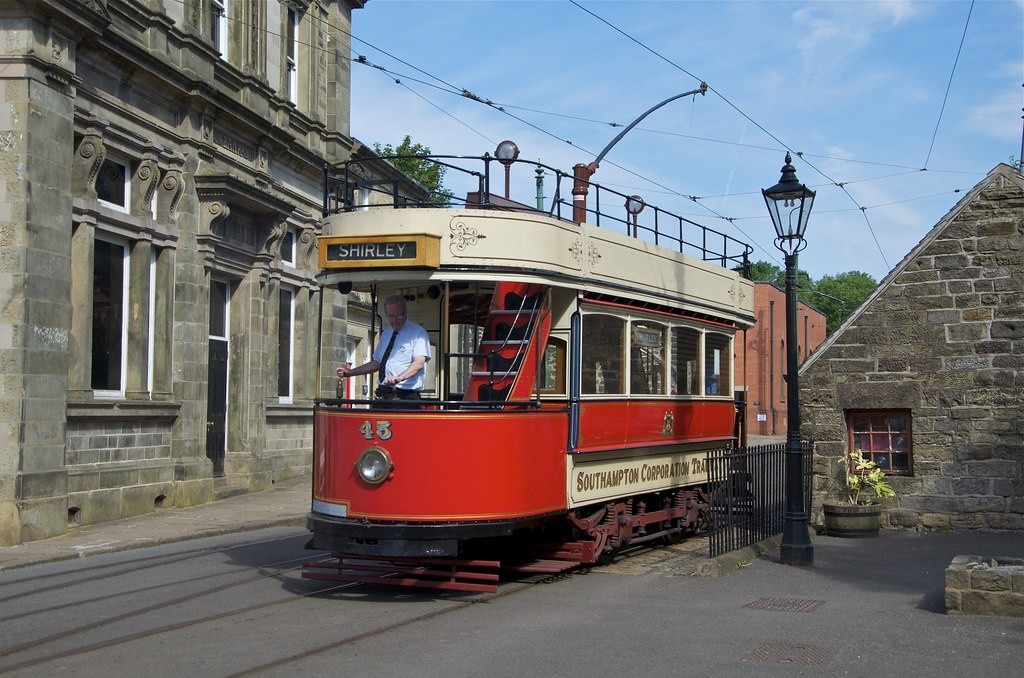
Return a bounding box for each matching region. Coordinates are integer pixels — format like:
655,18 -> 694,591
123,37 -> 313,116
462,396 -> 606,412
337,294 -> 431,410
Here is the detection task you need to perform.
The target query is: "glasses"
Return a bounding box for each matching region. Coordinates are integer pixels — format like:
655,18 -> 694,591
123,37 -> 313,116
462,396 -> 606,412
385,311 -> 405,320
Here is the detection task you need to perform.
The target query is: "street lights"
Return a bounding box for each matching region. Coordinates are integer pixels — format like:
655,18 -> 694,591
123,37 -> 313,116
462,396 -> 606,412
623,195 -> 645,236
760,152 -> 817,566
494,140 -> 519,199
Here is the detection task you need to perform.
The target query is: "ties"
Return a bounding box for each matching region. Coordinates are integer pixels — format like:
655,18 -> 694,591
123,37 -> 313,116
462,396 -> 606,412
378,330 -> 398,383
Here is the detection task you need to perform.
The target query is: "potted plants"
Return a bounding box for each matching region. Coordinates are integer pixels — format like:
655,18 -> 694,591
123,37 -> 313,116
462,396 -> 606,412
822,449 -> 896,538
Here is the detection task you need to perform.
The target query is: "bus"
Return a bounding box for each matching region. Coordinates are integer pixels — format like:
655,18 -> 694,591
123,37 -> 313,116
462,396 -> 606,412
298,152 -> 755,591
